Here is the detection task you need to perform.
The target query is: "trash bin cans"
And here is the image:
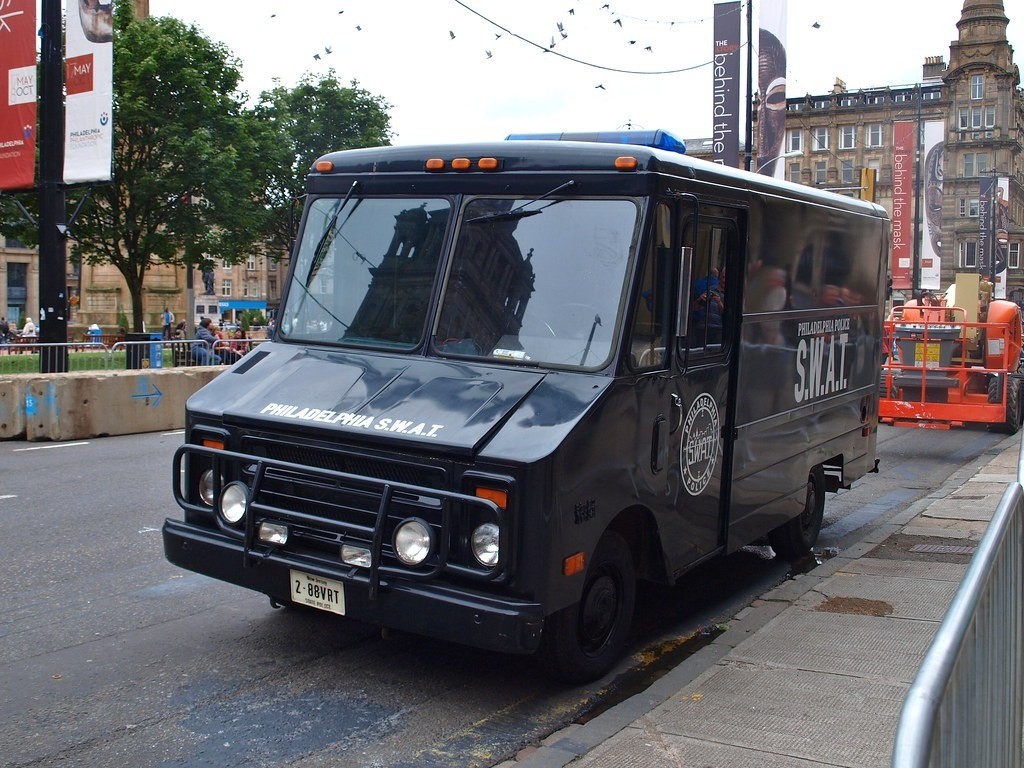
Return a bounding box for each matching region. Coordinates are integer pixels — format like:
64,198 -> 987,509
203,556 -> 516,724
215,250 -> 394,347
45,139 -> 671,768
87,329 -> 101,347
125,333 -> 163,370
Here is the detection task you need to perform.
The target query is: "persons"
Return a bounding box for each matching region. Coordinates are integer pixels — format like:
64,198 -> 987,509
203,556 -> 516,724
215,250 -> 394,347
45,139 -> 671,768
21,317 -> 37,336
694,265 -> 867,345
159,306 -> 175,340
0,317 -> 9,336
252,317 -> 261,331
176,319 -> 187,340
269,316 -> 331,333
190,317 -> 250,365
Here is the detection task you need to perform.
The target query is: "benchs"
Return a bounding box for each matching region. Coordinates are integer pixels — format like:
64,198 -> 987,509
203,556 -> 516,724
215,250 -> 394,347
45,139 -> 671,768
171,337 -> 197,367
73,333 -> 125,352
7,336 -> 40,355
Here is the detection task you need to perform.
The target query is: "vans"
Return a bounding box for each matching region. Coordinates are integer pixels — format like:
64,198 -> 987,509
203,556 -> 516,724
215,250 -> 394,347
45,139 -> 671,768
158,127 -> 896,692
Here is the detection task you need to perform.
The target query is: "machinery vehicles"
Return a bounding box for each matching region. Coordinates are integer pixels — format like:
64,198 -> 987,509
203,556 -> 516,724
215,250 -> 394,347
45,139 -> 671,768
878,273 -> 1024,435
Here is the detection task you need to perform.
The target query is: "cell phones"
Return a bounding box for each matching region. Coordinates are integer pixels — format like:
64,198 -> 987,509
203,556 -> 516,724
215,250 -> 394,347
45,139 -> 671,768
699,289 -> 714,307
